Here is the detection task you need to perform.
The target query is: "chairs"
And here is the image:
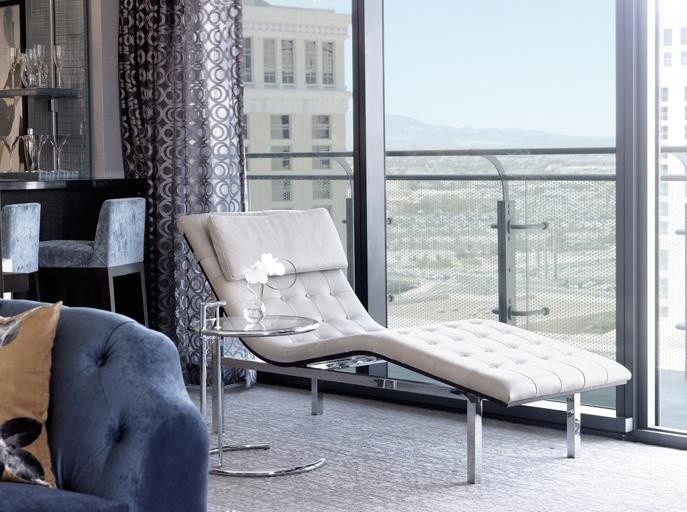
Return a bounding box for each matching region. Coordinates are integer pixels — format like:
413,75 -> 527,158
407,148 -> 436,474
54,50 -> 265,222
175,204 -> 633,484
1,198 -> 148,331
0,297 -> 208,511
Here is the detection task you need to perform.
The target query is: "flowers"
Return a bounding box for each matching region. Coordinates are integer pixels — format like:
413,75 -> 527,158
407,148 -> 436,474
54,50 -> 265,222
244,252 -> 286,285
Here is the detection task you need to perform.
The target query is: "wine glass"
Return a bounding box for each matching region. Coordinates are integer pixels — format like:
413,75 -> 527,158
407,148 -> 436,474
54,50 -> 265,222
0,134 -> 68,173
7,43 -> 64,89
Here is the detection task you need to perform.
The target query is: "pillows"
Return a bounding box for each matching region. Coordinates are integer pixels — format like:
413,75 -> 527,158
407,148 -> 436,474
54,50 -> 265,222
1,300 -> 63,491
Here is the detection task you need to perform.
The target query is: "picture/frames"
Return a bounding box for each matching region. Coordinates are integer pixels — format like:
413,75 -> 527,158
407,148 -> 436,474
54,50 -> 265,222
1,0 -> 29,180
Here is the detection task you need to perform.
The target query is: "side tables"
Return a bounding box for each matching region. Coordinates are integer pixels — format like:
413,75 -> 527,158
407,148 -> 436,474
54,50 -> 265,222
186,314 -> 327,477
